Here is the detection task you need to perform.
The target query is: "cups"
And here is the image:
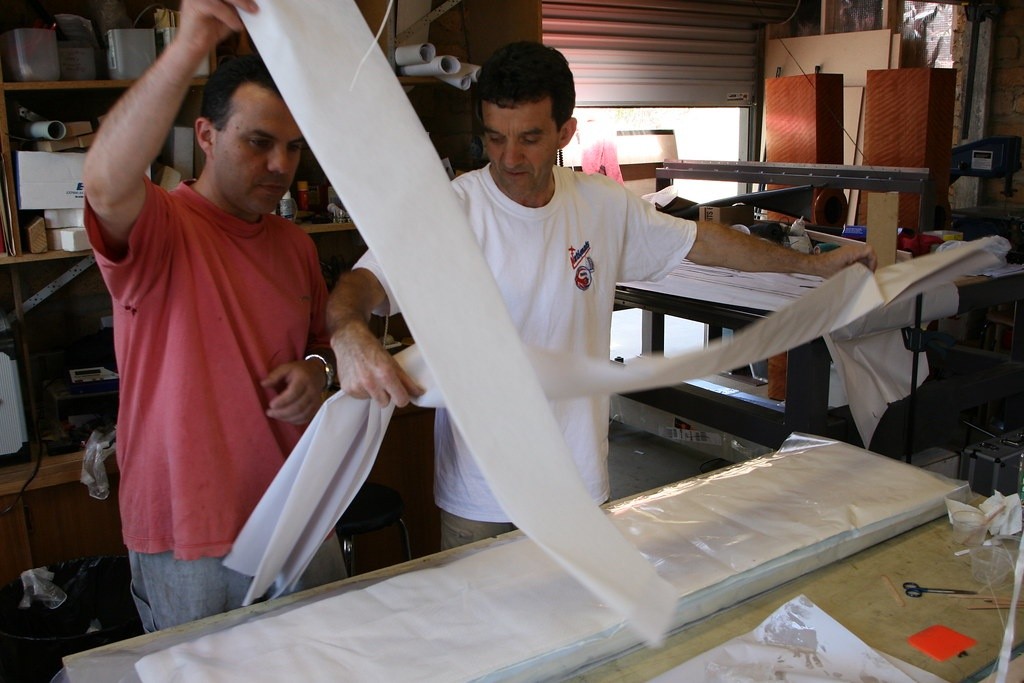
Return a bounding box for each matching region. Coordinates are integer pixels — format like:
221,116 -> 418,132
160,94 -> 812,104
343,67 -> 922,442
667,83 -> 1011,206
970,543 -> 1013,585
991,535 -> 1023,569
951,510 -> 989,548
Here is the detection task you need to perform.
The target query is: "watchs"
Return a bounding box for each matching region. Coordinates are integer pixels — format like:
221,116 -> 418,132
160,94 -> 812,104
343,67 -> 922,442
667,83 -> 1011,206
303,351 -> 336,394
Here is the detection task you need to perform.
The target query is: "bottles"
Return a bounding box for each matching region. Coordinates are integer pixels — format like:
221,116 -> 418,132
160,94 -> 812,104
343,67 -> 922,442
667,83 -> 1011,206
298,180 -> 309,211
281,190 -> 294,226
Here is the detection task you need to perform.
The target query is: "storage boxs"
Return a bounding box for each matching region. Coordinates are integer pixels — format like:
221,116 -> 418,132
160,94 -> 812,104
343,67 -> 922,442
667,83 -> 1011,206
158,24 -> 210,77
699,201 -> 755,225
173,126 -> 196,185
102,24 -> 157,82
151,166 -> 182,192
15,146 -> 150,210
5,25 -> 60,83
59,46 -> 96,82
36,111 -> 110,150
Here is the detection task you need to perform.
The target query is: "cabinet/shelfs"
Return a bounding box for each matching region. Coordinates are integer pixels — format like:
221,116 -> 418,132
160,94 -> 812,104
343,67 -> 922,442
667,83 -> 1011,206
1,1 -> 547,577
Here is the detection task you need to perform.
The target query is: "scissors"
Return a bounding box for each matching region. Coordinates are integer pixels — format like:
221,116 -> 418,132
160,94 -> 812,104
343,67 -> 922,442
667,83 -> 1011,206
903,582 -> 978,597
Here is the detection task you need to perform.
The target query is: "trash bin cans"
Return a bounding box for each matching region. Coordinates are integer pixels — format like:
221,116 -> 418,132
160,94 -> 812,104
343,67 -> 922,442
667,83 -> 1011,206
0,553 -> 138,682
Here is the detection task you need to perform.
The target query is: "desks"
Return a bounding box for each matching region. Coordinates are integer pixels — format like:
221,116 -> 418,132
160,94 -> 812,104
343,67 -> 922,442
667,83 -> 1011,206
47,437 -> 1024,683
611,232 -> 1024,471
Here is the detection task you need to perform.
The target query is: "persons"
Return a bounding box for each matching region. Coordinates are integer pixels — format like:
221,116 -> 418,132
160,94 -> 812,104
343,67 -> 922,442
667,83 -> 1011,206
78,1 -> 339,639
324,43 -> 879,553
